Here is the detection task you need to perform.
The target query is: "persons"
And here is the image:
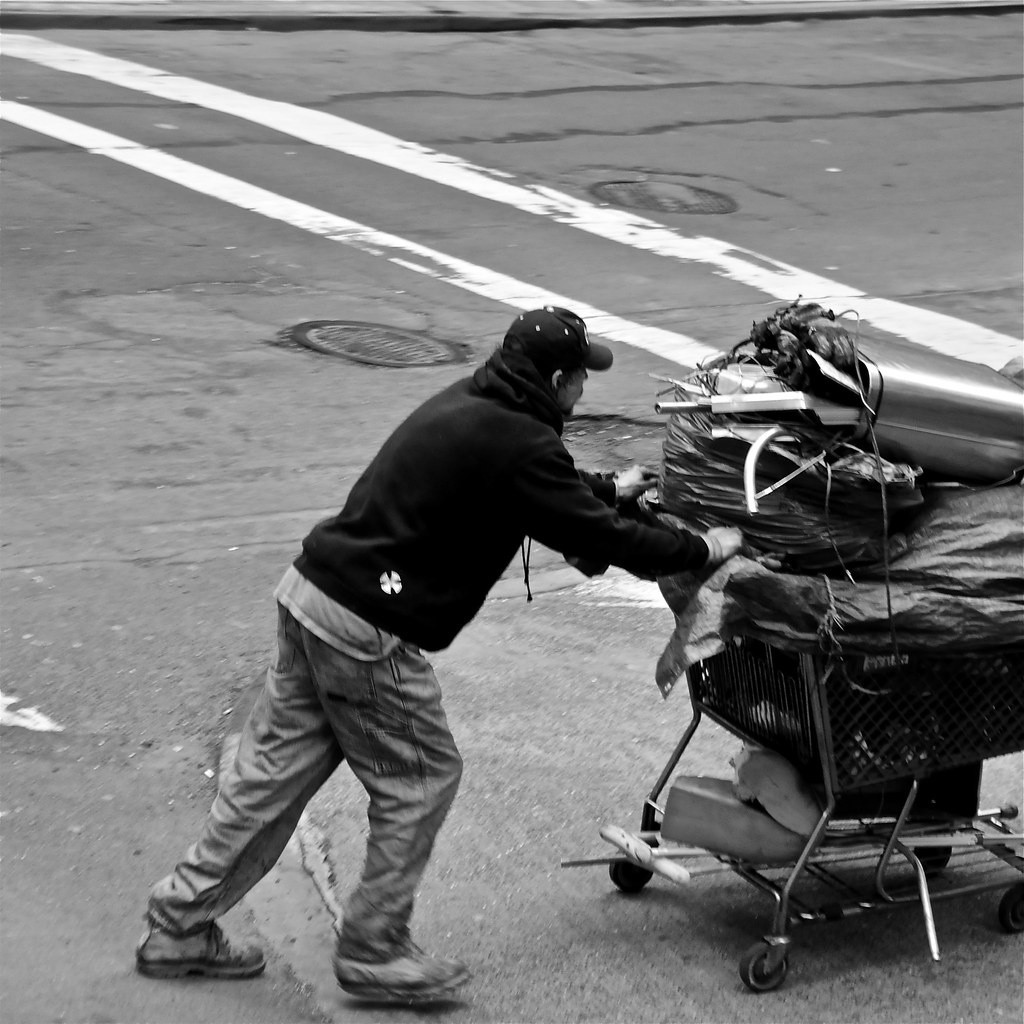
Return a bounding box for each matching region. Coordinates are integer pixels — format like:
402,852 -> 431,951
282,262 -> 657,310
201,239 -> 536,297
132,307 -> 758,1003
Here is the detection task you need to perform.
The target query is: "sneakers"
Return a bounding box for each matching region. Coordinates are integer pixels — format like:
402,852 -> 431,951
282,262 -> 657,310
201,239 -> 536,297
136,918 -> 267,982
328,942 -> 469,999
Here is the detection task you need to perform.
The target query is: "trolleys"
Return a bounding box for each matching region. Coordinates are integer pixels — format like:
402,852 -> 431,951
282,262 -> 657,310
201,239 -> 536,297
576,467 -> 1021,996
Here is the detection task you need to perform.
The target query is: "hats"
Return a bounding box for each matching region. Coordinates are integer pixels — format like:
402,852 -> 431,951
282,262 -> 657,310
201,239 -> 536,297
505,304 -> 614,372
